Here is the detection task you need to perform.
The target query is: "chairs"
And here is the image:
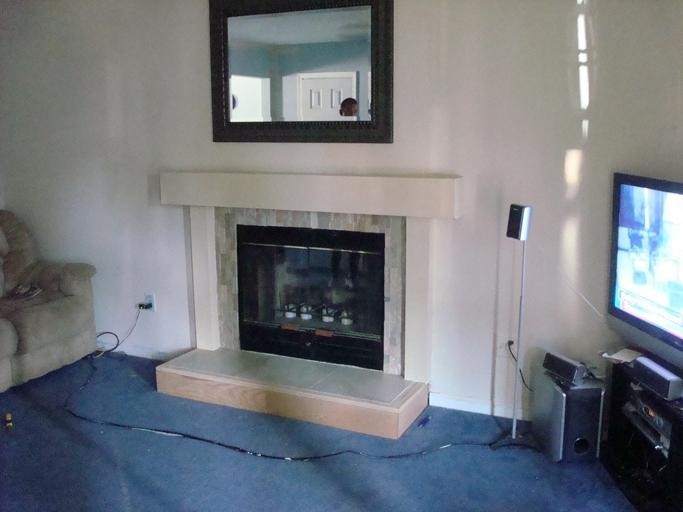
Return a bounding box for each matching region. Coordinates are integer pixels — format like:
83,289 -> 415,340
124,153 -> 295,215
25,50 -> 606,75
0,209 -> 98,395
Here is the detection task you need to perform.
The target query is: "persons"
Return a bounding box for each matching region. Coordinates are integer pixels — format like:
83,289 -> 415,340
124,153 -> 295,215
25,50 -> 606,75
338,97 -> 359,115
330,249 -> 359,282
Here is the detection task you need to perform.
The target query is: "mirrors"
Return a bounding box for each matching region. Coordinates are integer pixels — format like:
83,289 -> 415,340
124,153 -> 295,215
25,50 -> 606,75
209,0 -> 394,145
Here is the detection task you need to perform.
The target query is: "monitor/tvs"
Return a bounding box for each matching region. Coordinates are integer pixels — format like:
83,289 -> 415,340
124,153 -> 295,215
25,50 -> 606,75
608,173 -> 683,351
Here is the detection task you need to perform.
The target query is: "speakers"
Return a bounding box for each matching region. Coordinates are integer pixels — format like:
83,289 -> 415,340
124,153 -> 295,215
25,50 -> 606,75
543,352 -> 586,385
506,204 -> 531,240
531,359 -> 605,463
633,357 -> 683,401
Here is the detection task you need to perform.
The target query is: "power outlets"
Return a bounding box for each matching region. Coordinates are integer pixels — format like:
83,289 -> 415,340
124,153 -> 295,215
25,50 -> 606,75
506,336 -> 515,358
144,293 -> 155,312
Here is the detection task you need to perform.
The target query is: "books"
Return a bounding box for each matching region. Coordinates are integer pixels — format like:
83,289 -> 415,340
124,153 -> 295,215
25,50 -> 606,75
3,284 -> 43,304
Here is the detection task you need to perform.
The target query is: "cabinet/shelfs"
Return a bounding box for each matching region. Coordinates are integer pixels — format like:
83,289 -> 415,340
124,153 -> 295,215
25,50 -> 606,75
282,72 -> 356,121
598,361 -> 683,512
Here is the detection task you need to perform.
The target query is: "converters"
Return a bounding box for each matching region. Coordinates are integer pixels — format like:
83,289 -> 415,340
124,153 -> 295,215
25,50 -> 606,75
139,302 -> 152,309
